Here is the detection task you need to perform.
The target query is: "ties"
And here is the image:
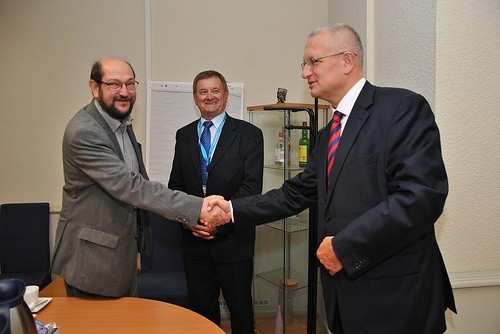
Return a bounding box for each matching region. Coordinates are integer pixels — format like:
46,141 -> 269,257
199,121 -> 214,195
328,110 -> 343,176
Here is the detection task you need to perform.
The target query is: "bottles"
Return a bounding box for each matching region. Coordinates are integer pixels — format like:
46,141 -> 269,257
274,305 -> 286,334
299,122 -> 309,167
275,131 -> 291,167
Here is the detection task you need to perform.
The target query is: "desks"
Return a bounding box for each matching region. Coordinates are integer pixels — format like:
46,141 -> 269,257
33,298 -> 225,334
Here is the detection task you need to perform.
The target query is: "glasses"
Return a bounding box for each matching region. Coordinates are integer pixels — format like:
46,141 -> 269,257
95,80 -> 139,91
301,50 -> 358,71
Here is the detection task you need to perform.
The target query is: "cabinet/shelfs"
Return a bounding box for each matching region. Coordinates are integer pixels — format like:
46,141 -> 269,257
245,103 -> 331,334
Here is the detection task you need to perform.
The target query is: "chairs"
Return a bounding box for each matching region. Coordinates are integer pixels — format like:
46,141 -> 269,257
0,203 -> 51,297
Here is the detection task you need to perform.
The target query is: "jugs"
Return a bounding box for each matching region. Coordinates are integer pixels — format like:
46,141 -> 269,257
0,278 -> 39,334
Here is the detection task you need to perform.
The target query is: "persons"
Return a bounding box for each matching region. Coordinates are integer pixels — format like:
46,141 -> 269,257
48,57 -> 230,299
199,23 -> 458,334
168,70 -> 264,334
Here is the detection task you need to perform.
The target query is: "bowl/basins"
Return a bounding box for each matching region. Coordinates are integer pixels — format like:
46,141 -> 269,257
23,285 -> 39,310
